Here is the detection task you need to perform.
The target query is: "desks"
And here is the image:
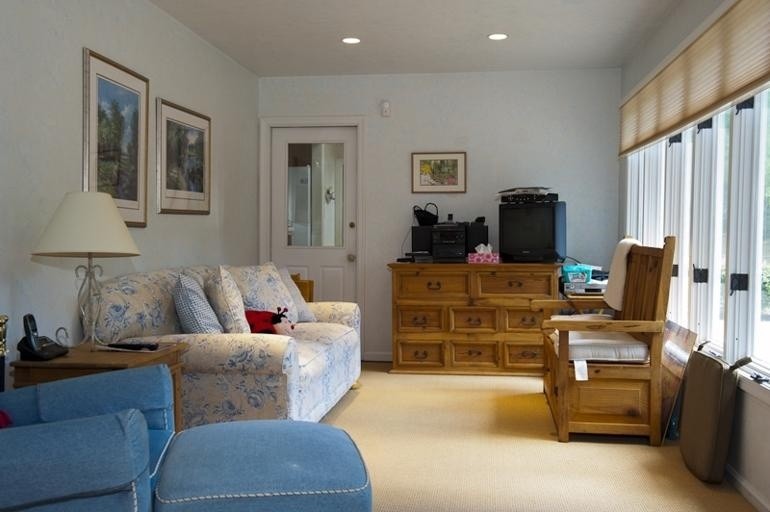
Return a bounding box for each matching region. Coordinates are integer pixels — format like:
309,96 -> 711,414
565,292 -> 604,314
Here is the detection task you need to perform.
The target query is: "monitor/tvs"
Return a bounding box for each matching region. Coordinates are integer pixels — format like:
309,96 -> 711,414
497,201 -> 567,264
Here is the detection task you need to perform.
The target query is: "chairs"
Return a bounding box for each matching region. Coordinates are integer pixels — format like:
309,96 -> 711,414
0,365 -> 175,511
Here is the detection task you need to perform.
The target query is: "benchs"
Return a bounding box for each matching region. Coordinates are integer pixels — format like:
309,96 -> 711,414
532,236 -> 678,445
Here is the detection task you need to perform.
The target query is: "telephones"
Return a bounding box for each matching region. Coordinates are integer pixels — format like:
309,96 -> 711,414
17,314 -> 69,361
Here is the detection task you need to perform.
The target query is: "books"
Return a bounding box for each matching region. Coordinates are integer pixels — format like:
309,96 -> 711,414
95,343 -> 176,352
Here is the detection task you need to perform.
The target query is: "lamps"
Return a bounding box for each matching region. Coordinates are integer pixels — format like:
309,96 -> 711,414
29,190 -> 142,352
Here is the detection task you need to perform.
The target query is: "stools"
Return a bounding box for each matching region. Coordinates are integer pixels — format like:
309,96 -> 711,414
150,420 -> 375,511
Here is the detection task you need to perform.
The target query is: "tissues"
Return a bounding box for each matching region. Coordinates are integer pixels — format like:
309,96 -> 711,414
468,244 -> 500,263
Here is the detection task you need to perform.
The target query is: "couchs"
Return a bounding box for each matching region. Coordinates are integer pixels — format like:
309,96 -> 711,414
84,259 -> 360,421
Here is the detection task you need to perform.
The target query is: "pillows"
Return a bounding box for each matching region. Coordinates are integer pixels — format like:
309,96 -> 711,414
174,269 -> 225,332
204,266 -> 251,332
276,265 -> 316,323
227,262 -> 298,323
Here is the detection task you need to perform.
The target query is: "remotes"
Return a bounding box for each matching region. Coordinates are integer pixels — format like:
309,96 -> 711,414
109,343 -> 160,350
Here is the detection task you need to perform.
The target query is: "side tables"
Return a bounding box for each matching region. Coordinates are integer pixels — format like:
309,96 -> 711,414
6,343 -> 188,432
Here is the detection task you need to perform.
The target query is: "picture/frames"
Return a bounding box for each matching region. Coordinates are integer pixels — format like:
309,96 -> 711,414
155,97 -> 210,215
410,150 -> 467,194
84,46 -> 150,227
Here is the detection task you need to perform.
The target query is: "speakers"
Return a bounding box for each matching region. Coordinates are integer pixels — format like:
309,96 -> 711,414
411,225 -> 432,253
466,225 -> 489,253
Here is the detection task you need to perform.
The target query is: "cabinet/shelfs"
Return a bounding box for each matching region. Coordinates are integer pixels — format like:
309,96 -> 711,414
386,260 -> 563,374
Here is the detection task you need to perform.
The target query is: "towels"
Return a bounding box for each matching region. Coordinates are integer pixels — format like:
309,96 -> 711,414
602,239 -> 642,310
